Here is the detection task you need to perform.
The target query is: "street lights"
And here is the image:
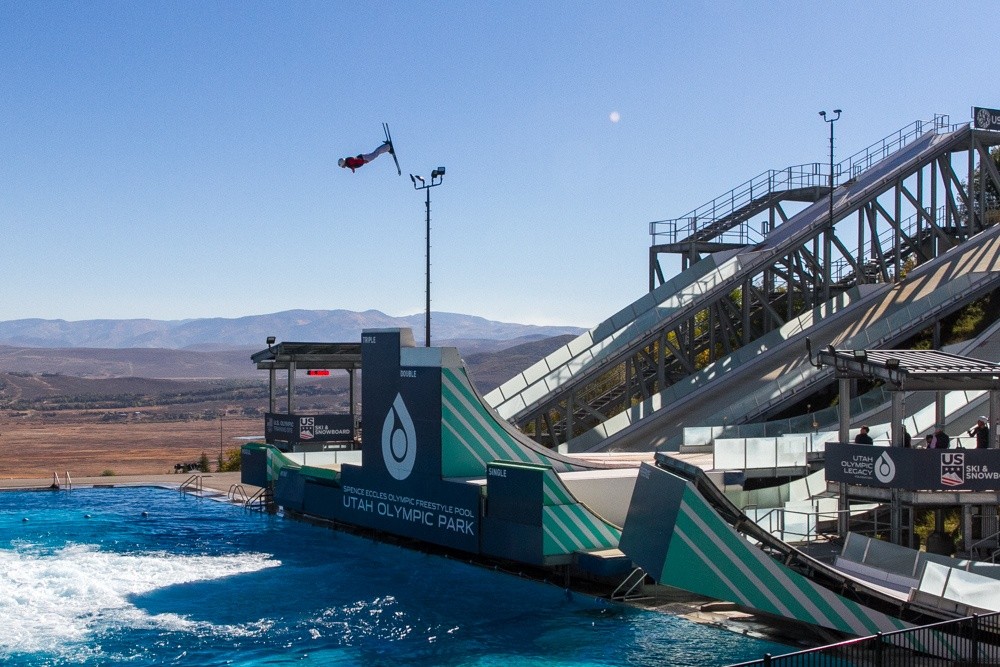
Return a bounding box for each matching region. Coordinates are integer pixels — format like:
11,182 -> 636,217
818,109 -> 843,228
409,166 -> 447,349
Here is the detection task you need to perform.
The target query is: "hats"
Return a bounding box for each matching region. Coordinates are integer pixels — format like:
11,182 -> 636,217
977,416 -> 988,423
933,424 -> 944,428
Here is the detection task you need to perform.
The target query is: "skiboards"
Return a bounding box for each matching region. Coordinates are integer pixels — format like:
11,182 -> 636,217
382,122 -> 402,176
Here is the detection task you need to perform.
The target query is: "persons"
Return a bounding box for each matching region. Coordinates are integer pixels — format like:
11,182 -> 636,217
338,140 -> 394,174
902,425 -> 911,448
855,425 -> 873,444
968,415 -> 989,448
926,423 -> 949,449
